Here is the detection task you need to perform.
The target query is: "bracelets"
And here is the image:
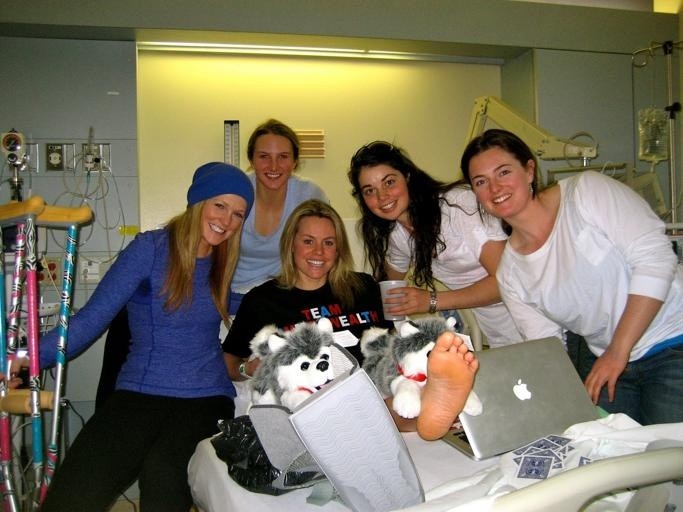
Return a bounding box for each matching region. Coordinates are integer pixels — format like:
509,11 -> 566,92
428,289 -> 439,314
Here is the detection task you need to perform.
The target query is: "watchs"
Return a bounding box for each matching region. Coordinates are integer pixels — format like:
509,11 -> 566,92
238,363 -> 253,380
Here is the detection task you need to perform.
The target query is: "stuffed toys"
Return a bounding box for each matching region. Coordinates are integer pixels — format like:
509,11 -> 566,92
360,316 -> 463,420
248,317 -> 359,411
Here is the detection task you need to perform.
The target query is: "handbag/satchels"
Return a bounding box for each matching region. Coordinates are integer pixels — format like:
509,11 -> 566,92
211,415 -> 327,496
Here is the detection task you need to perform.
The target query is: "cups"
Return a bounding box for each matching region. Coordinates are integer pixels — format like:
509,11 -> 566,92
379,280 -> 409,321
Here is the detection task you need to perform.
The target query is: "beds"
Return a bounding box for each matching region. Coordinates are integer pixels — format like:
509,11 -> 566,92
189,265 -> 683,512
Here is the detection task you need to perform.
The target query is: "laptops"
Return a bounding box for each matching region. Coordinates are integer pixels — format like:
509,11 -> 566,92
439,337 -> 601,461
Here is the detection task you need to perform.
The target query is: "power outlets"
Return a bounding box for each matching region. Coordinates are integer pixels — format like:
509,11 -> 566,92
82,145 -> 103,172
78,259 -> 100,285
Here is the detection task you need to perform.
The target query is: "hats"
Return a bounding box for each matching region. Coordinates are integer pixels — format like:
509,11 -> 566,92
187,162 -> 254,220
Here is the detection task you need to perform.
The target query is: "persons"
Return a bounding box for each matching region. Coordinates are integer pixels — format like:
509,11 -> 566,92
225,117 -> 331,314
0,161 -> 255,512
460,128 -> 682,426
216,198 -> 480,441
346,140 -> 568,357
